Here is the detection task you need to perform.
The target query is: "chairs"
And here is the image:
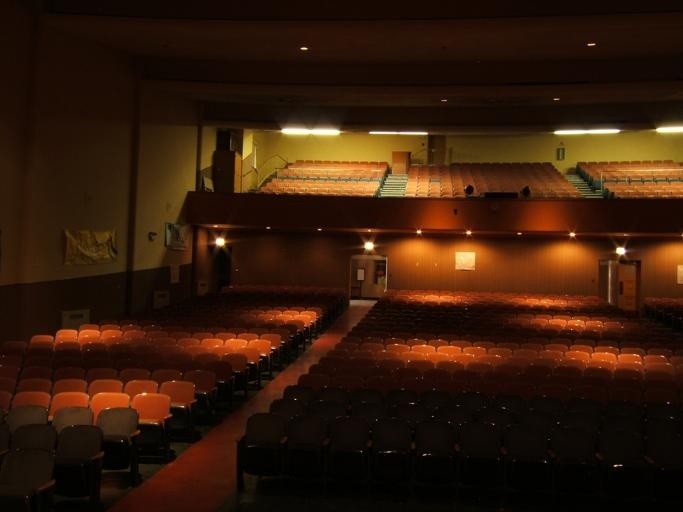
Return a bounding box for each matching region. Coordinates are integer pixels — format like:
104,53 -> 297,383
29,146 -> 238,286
405,161 -> 683,198
0,287 -> 683,512
256,160 -> 389,197
